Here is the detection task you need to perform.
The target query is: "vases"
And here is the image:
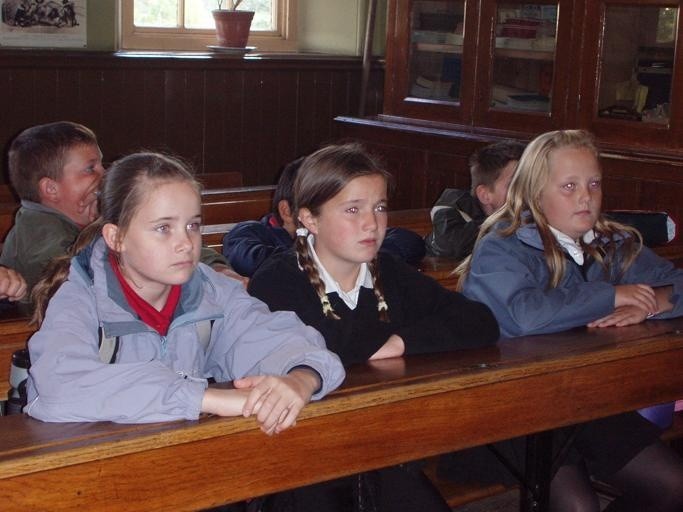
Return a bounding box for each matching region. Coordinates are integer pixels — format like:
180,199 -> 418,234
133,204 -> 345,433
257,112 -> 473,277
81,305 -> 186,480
212,9 -> 255,47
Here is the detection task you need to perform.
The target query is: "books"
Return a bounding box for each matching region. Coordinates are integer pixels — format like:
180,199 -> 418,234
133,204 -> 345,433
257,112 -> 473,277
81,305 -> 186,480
406,17 -> 557,113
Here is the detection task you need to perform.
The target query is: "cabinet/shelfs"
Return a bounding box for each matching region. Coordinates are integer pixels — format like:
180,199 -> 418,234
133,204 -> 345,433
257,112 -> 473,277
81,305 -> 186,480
382,0 -> 587,142
577,0 -> 683,164
333,116 -> 471,209
601,154 -> 682,270
0,52 -> 383,188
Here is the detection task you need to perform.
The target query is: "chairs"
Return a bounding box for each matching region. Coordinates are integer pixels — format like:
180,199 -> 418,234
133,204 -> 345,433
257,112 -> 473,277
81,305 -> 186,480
2,171 -> 683,511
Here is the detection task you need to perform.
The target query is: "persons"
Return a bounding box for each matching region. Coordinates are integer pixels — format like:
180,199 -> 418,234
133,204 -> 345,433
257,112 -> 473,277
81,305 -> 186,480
421,135 -> 526,267
13,0 -> 78,29
225,153 -> 428,276
20,149 -> 346,436
459,127 -> 681,511
244,134 -> 502,512
0,265 -> 29,302
1,117 -> 105,309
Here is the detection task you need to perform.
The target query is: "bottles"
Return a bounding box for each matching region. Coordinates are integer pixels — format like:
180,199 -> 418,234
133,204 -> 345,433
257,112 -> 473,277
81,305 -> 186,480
9,348 -> 31,398
